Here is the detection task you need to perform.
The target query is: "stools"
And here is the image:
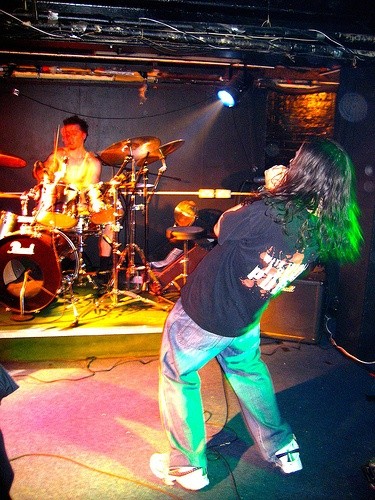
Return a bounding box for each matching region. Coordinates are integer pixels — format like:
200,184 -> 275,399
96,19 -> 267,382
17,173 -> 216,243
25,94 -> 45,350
162,226 -> 205,293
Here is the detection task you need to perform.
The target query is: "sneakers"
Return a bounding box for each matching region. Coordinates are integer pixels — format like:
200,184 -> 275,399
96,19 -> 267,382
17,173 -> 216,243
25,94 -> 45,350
150,453 -> 209,491
276,435 -> 303,474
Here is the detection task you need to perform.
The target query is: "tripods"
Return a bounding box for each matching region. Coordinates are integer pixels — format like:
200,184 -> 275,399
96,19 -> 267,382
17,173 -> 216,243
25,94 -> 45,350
64,163 -> 171,327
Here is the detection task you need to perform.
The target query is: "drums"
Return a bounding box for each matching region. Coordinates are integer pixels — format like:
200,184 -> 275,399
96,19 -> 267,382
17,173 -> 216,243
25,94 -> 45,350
33,181 -> 82,230
0,225 -> 79,313
62,214 -> 103,234
0,210 -> 23,235
85,183 -> 125,226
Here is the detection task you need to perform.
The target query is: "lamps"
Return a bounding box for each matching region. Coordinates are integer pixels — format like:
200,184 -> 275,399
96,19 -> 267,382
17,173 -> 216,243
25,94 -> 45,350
217,66 -> 254,107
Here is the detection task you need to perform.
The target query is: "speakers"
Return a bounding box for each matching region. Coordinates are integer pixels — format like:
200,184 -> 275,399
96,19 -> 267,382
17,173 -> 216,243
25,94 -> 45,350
261,280 -> 324,342
157,245 -> 211,291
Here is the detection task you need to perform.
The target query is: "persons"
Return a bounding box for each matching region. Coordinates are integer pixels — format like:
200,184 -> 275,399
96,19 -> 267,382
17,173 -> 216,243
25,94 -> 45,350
43,115 -> 114,296
148,137 -> 363,491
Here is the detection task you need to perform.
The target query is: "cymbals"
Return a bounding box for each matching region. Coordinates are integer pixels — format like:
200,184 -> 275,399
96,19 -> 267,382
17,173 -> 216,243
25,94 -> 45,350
136,140 -> 185,168
0,154 -> 27,168
101,180 -> 155,190
101,136 -> 160,166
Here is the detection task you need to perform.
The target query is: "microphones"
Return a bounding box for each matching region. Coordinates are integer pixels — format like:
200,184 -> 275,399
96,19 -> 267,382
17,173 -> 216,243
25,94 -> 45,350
252,169 -> 289,182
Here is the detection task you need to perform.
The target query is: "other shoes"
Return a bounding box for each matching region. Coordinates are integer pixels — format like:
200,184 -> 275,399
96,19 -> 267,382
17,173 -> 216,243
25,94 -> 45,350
95,274 -> 108,290
